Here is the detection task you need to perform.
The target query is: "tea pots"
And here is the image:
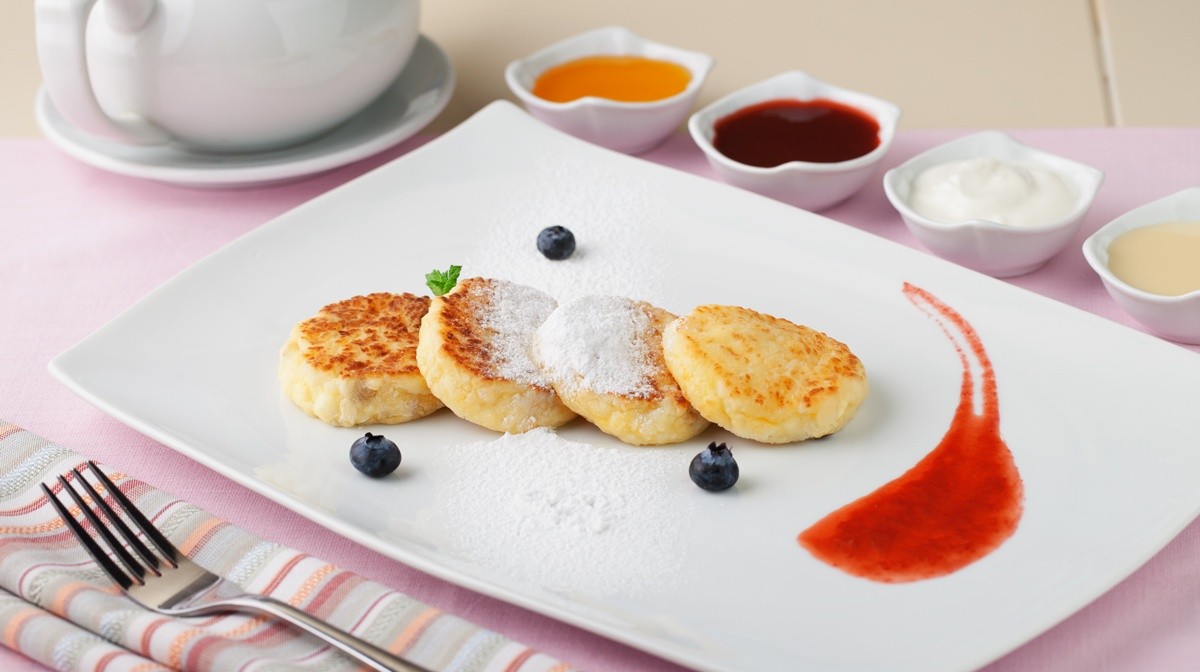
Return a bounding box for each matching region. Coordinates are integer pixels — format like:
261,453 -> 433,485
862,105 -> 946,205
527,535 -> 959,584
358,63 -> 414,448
31,1 -> 422,155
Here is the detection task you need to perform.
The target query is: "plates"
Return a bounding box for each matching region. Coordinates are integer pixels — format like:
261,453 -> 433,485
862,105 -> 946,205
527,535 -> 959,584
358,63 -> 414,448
48,98 -> 1199,672
33,32 -> 455,188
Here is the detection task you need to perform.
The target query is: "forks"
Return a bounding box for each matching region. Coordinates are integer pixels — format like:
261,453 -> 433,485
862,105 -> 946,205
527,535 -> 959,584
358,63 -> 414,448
40,459 -> 437,672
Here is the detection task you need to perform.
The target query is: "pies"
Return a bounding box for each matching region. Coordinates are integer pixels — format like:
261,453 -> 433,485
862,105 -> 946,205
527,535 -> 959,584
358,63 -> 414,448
277,277 -> 867,448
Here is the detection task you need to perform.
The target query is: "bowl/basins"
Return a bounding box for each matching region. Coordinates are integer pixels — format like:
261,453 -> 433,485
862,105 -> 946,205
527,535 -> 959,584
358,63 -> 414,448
1082,185 -> 1198,344
882,129 -> 1104,279
687,69 -> 900,212
503,24 -> 713,155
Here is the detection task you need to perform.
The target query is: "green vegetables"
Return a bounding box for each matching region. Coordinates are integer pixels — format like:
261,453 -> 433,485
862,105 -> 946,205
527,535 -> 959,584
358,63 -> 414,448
425,266 -> 463,297
